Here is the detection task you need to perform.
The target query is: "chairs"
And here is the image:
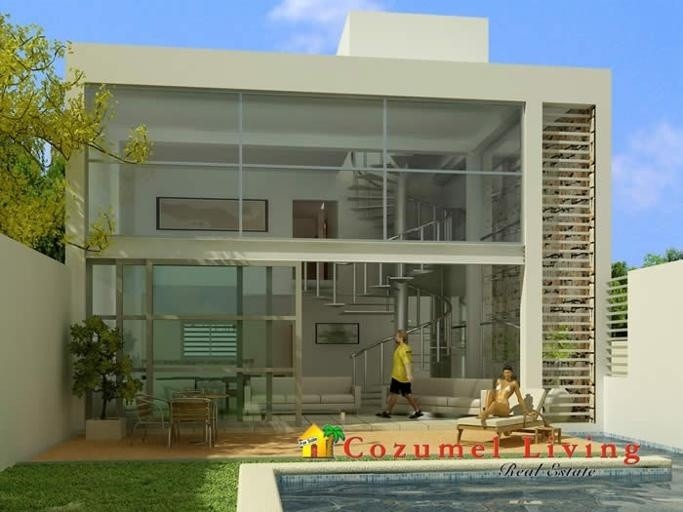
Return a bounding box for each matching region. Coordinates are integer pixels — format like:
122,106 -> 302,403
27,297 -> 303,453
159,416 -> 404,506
456,387 -> 545,446
130,388 -> 211,448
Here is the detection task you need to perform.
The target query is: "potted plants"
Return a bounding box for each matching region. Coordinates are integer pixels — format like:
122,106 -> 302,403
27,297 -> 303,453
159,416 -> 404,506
69,316 -> 143,442
542,326 -> 578,422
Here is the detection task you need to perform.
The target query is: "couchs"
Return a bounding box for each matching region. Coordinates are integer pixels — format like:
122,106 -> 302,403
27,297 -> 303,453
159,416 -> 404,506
381,377 -> 494,415
244,376 -> 361,415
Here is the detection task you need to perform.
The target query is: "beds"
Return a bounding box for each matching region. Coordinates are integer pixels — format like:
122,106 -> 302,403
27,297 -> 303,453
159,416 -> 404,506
162,393 -> 229,447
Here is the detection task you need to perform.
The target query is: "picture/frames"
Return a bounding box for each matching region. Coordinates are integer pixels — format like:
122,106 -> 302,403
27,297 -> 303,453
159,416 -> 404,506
156,196 -> 268,232
315,323 -> 359,344
